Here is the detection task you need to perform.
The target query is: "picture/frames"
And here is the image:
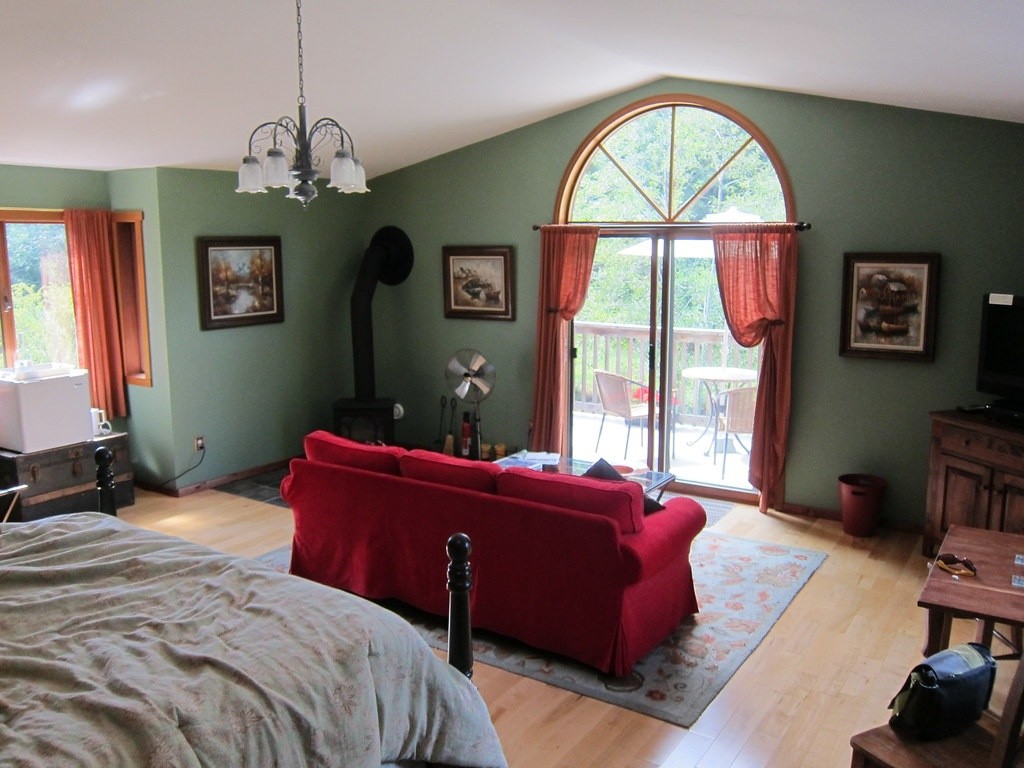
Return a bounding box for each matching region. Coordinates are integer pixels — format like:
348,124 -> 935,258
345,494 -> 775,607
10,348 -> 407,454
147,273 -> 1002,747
440,245 -> 516,322
839,250 -> 940,362
196,233 -> 285,331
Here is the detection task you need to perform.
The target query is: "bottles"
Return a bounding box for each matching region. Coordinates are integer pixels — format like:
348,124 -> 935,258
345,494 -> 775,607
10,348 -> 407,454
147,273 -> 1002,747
14,334 -> 34,380
481,444 -> 491,459
496,444 -> 505,460
461,412 -> 472,456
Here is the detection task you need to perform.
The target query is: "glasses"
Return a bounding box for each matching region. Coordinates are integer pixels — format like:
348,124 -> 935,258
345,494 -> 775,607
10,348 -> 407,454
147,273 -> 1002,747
936,552 -> 978,578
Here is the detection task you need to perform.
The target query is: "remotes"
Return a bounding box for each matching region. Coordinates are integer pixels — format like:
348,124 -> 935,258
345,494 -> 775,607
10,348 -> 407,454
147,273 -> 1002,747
957,404 -> 986,414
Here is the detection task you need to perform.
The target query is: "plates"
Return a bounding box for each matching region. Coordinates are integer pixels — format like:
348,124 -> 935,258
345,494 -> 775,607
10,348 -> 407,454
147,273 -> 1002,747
613,465 -> 634,475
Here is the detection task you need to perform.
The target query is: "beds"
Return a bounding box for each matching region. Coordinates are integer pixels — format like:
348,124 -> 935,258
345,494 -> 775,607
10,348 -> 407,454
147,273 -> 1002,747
0,447 -> 506,768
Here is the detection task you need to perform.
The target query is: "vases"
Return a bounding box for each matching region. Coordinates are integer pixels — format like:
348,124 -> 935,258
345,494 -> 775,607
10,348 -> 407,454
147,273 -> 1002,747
625,416 -> 660,429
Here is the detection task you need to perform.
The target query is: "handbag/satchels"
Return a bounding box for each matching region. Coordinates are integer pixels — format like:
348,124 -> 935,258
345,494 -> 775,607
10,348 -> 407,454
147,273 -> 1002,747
886,642 -> 997,744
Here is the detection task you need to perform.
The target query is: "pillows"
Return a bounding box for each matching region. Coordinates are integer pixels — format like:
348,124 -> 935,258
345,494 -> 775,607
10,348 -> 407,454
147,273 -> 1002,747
579,458 -> 669,514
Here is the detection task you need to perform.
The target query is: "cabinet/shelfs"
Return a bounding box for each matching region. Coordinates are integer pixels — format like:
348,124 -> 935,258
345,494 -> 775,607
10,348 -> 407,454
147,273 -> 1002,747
923,410 -> 1024,563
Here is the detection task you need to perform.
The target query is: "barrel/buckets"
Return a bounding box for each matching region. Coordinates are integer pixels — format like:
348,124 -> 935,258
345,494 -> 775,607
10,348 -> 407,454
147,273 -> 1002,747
838,473 -> 887,537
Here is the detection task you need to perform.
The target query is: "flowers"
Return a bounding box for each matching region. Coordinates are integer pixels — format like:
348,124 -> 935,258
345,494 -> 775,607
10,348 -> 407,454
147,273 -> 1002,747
632,386 -> 678,406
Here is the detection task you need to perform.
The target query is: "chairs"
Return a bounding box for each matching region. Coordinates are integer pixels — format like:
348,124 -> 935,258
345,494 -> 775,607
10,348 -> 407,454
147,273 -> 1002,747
713,385 -> 758,478
850,659 -> 1024,768
593,370 -> 680,460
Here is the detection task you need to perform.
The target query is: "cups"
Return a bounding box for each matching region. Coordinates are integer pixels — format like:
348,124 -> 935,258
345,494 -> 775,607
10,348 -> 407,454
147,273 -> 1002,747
90,408 -> 105,435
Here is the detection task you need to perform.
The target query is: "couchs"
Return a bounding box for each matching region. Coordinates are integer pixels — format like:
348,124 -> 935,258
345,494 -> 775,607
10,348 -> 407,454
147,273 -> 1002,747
279,431 -> 706,693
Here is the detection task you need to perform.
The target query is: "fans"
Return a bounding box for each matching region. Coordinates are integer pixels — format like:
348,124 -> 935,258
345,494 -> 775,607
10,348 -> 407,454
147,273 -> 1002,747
445,349 -> 497,459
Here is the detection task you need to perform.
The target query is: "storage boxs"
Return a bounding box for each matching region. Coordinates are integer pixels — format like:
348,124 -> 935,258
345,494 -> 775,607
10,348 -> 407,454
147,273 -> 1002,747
0,367 -> 134,522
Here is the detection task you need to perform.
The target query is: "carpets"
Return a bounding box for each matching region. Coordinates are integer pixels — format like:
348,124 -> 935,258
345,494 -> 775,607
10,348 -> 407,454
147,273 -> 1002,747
649,490 -> 737,529
252,525 -> 828,728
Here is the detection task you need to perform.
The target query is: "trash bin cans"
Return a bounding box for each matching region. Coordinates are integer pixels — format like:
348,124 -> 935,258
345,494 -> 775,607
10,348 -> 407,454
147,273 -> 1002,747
838,474 -> 888,538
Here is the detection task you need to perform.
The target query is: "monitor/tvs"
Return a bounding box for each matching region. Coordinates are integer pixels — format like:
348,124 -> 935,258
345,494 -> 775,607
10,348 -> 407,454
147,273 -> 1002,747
976,292 -> 1024,418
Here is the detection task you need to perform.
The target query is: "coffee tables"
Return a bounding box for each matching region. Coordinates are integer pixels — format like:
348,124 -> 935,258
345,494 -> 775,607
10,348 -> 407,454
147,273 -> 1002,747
494,449 -> 676,502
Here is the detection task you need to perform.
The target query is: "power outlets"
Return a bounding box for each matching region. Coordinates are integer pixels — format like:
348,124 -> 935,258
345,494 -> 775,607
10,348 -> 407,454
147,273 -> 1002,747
194,435 -> 205,452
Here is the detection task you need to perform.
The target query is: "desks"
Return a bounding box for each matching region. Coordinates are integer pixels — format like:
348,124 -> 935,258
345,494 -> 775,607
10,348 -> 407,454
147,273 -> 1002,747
917,523 -> 1023,661
681,367 -> 758,456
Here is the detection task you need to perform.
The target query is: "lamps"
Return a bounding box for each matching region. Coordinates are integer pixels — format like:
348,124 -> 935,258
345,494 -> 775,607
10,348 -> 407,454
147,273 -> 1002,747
235,0 -> 371,209
